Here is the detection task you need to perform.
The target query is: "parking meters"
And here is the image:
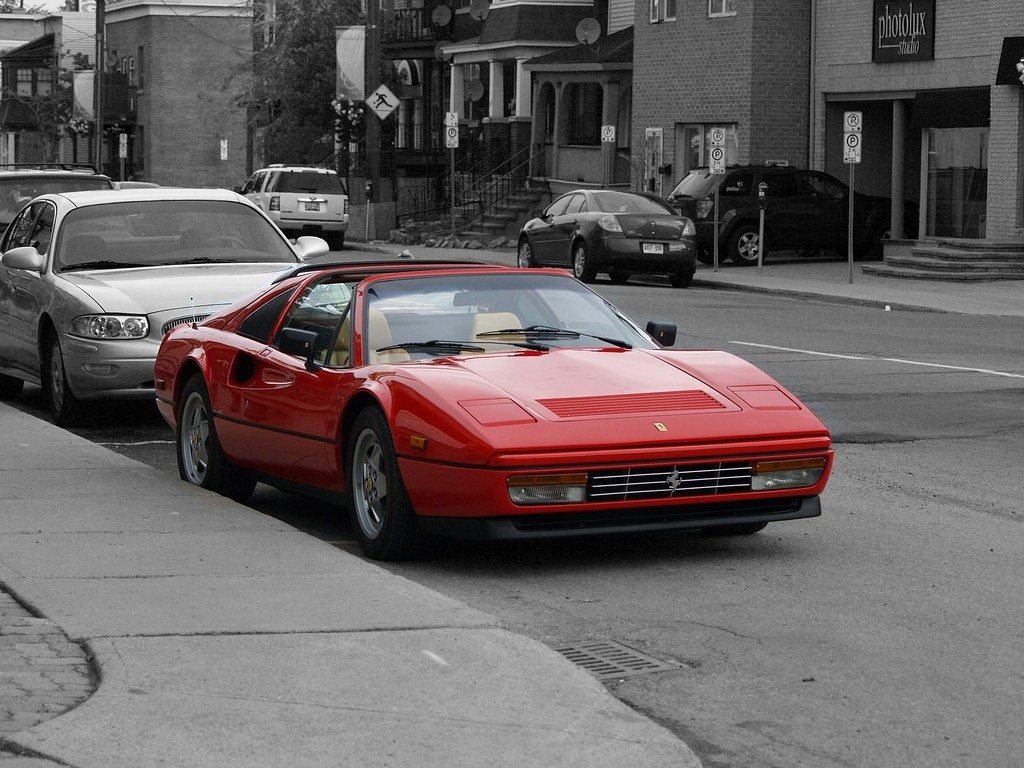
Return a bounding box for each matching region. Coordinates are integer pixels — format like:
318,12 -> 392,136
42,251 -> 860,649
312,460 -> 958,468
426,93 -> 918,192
757,182 -> 770,272
365,180 -> 373,242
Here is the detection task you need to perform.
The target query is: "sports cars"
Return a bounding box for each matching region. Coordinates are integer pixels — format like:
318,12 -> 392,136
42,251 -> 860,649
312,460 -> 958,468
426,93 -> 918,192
156,260 -> 834,561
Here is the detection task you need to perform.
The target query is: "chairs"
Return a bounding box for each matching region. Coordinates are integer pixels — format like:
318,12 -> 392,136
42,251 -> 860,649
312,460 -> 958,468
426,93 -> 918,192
319,309 -> 410,366
459,312 -> 526,355
64,235 -> 107,265
180,228 -> 223,250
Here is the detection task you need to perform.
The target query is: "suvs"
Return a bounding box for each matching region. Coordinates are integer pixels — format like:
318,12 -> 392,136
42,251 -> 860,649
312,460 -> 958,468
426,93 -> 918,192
666,160 -> 919,266
234,165 -> 350,250
927,165 -> 987,236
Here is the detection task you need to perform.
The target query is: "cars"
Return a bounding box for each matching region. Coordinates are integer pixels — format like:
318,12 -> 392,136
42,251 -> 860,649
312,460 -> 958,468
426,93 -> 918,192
517,190 -> 697,288
0,161 -> 331,427
